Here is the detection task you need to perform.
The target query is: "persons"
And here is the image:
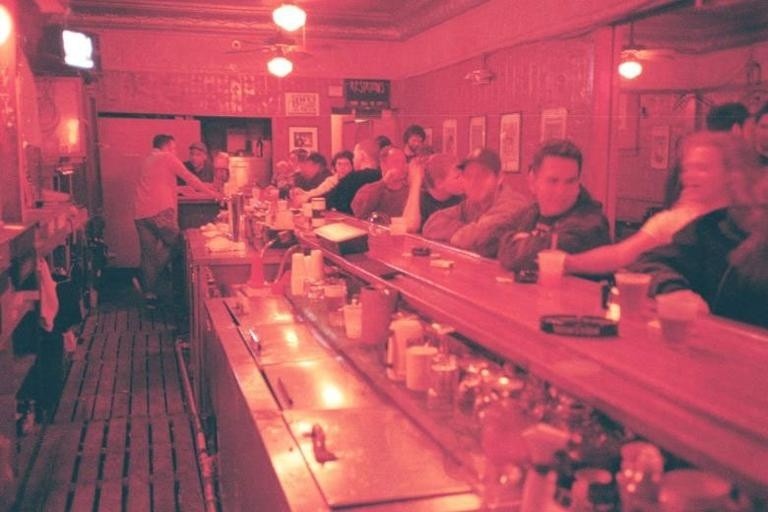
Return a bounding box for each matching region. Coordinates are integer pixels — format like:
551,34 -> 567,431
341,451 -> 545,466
482,136 -> 611,281
422,147 -> 536,261
280,124 -> 434,229
537,102 -> 767,326
131,133 -> 215,311
176,142 -> 213,186
402,154 -> 466,234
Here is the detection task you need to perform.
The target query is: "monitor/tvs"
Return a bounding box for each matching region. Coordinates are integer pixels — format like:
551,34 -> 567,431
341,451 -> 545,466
42,24 -> 100,74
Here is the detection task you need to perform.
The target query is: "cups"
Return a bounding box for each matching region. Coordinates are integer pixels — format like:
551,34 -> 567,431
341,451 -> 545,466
223,179 -> 755,510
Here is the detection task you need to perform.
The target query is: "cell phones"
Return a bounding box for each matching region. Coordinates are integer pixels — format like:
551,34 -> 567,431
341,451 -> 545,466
540,314 -> 618,337
412,248 -> 429,256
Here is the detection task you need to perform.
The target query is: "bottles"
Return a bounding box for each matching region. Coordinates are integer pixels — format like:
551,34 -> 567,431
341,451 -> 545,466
245,135 -> 252,155
256,137 -> 263,158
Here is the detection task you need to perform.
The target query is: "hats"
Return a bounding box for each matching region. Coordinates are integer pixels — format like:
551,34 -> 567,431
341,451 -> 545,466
456,146 -> 501,177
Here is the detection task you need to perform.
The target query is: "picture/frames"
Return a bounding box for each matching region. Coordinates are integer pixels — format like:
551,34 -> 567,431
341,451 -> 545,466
496,110 -> 523,174
539,107 -> 569,143
288,125 -> 319,156
439,116 -> 459,159
467,114 -> 487,152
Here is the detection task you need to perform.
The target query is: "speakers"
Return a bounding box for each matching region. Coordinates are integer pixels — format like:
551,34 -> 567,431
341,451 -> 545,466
343,79 -> 391,101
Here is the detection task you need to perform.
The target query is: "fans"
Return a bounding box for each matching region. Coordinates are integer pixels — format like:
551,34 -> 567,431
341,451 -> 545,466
223,23 -> 338,72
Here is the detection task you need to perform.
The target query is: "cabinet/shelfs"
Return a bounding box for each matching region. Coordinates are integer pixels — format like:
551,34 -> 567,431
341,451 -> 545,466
0,220 -> 46,477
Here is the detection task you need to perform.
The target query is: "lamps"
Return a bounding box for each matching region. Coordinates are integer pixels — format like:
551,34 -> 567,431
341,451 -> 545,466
618,17 -> 643,79
272,1 -> 306,32
465,54 -> 495,85
267,48 -> 294,78
